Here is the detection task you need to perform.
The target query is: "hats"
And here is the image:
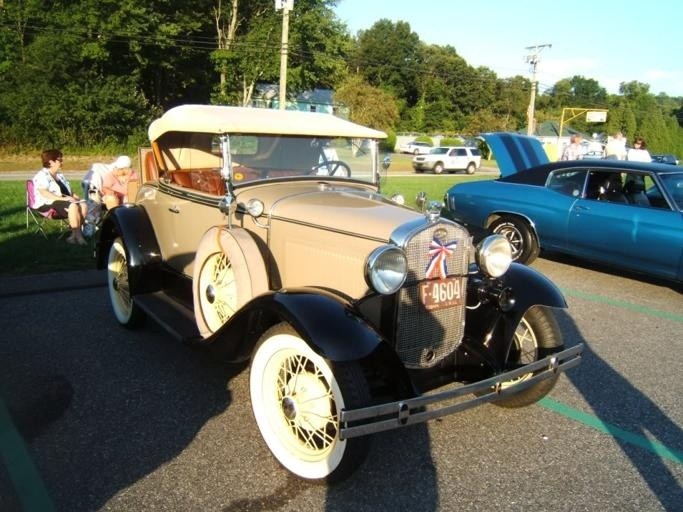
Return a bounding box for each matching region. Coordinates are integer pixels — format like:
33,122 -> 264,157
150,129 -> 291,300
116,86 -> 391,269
112,156 -> 130,169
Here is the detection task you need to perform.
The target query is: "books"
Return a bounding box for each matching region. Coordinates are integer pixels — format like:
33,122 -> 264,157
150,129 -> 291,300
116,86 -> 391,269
69,199 -> 87,203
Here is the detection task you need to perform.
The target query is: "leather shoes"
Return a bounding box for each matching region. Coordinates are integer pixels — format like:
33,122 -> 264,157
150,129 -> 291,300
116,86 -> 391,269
75,231 -> 88,248
65,235 -> 77,245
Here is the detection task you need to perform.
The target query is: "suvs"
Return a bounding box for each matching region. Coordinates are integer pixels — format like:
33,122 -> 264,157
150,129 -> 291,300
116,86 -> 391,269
409,146 -> 482,174
70,98 -> 585,484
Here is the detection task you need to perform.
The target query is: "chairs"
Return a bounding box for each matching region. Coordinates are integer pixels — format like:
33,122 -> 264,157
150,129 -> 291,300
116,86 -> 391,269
24,180 -> 76,242
169,166 -> 259,205
598,178 -> 630,208
266,168 -> 316,179
623,179 -> 652,209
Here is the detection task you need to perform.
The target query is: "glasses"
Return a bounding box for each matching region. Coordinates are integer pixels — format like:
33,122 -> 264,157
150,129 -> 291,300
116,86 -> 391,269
56,158 -> 62,162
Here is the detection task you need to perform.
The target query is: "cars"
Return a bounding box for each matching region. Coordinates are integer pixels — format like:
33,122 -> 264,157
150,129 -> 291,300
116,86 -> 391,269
438,131 -> 682,289
648,153 -> 678,165
579,148 -> 602,158
400,139 -> 432,155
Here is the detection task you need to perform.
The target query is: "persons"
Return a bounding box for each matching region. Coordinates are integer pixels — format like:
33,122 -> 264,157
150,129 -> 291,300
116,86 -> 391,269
605,131 -> 627,160
628,137 -> 652,162
32,149 -> 88,247
101,156 -> 137,210
562,134 -> 582,161
